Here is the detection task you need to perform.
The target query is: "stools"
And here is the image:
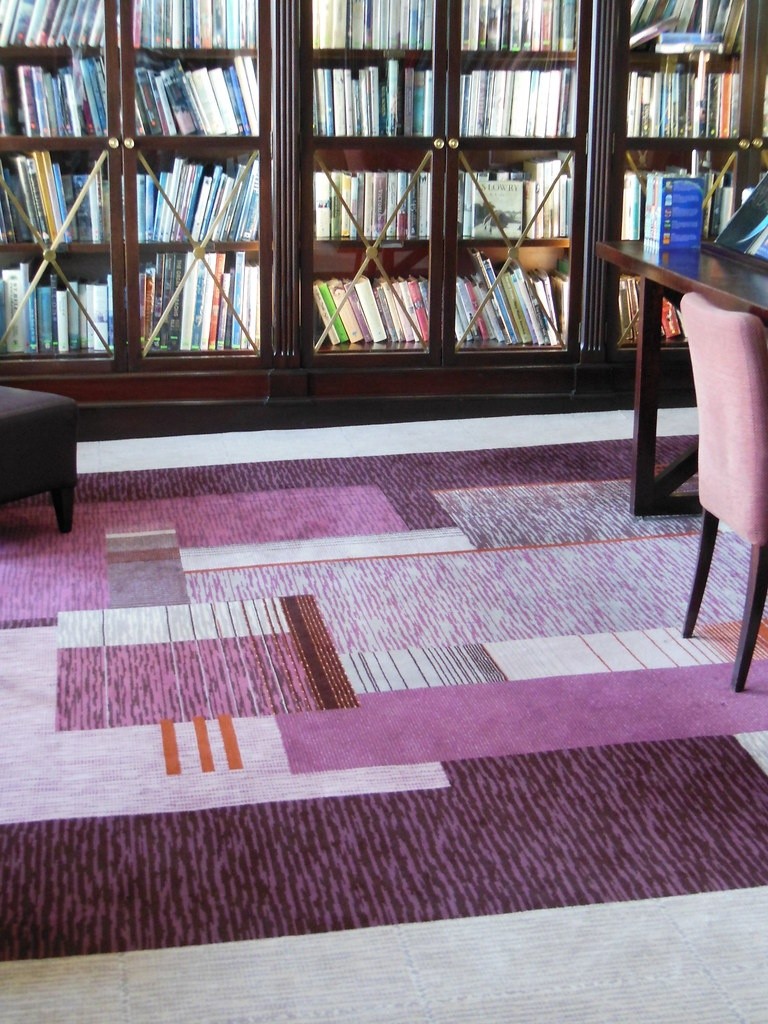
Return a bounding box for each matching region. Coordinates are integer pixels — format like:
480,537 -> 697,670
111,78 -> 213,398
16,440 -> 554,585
0,385 -> 76,534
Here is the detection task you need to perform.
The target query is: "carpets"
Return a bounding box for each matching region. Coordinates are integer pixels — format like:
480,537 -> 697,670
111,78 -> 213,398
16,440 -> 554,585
0,436 -> 768,1024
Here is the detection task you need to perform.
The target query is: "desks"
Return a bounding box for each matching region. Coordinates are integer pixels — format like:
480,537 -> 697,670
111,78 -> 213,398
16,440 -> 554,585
595,239 -> 768,517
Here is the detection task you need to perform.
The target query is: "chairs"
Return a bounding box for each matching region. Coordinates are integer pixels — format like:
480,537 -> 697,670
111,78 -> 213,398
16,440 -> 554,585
680,293 -> 768,693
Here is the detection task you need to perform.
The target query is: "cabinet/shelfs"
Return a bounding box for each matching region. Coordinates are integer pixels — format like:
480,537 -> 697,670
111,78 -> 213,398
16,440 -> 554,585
0,0 -> 768,441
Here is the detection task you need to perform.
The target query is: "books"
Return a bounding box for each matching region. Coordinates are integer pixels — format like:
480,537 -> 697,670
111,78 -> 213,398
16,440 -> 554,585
620,0 -> 768,345
137,0 -> 261,353
312,0 -> 433,346
0,0 -> 113,353
456,0 -> 578,346
